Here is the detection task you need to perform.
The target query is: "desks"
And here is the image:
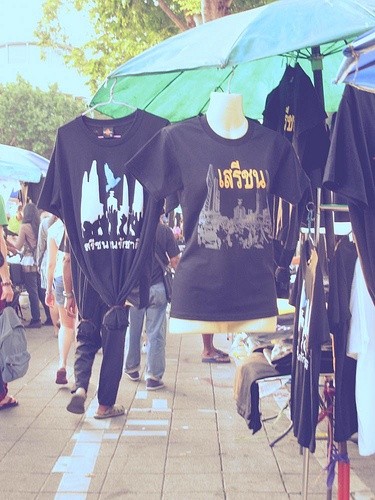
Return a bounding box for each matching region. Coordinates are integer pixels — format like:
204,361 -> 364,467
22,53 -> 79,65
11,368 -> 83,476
230,350 -> 358,448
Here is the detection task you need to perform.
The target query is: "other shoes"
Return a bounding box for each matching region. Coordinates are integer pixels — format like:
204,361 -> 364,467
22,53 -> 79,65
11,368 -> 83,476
56,368 -> 67,384
43,319 -> 53,326
25,319 -> 42,328
56,321 -> 60,328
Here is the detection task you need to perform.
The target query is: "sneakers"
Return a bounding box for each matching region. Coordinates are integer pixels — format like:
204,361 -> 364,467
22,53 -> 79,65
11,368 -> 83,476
124,368 -> 139,380
147,379 -> 165,391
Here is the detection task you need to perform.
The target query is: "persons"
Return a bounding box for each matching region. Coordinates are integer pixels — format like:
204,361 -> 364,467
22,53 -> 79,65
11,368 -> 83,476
0,197 -> 230,420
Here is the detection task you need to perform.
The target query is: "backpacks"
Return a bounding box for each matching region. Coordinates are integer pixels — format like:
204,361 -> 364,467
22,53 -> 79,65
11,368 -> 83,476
0,298 -> 30,384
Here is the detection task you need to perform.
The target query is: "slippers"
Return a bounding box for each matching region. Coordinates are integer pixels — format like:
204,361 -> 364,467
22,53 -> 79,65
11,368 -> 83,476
0,397 -> 18,410
201,348 -> 230,363
67,393 -> 86,413
93,404 -> 125,418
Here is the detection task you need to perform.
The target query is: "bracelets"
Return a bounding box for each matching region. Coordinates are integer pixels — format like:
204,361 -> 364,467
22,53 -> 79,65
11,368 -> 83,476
45,292 -> 52,296
1,280 -> 11,287
63,291 -> 74,297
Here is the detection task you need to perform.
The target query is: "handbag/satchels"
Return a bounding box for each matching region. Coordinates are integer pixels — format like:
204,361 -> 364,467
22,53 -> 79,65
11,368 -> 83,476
163,267 -> 173,301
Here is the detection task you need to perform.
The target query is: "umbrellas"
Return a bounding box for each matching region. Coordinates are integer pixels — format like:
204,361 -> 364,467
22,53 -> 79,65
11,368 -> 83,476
0,142 -> 50,186
87,0 -> 375,267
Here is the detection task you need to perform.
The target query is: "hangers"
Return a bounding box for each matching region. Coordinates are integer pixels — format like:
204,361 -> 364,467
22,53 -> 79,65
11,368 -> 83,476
347,230 -> 352,236
304,210 -> 316,246
81,74 -> 136,115
280,49 -> 301,83
